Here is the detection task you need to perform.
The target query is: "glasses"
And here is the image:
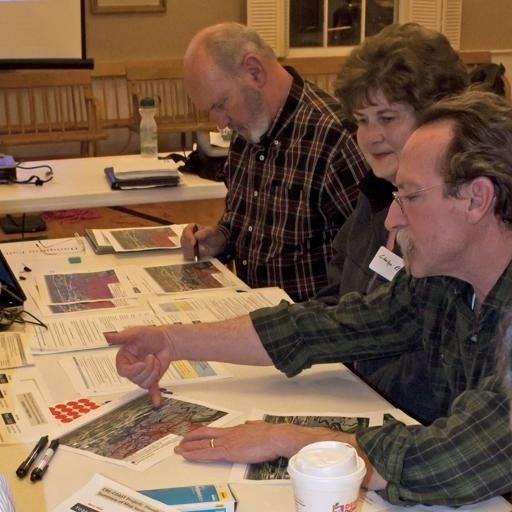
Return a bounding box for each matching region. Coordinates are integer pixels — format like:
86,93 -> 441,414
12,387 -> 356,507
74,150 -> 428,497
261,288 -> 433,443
392,183 -> 443,214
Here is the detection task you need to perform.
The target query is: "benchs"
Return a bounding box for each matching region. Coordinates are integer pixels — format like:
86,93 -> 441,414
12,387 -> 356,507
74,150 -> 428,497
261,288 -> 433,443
0,50 -> 491,171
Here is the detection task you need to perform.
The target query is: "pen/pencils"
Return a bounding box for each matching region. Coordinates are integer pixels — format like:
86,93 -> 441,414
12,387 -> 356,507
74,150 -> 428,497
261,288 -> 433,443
15,435 -> 60,482
193,223 -> 199,264
73,231 -> 86,251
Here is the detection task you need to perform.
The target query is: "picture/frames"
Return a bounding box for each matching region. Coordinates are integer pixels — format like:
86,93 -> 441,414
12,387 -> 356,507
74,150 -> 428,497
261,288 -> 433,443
90,0 -> 166,14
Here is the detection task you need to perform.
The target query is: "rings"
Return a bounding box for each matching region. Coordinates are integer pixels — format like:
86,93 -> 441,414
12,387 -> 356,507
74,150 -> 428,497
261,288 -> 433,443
210,437 -> 215,447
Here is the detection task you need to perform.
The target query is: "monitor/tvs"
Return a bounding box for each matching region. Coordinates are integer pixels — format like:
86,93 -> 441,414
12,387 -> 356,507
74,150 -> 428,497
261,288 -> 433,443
0,250 -> 27,308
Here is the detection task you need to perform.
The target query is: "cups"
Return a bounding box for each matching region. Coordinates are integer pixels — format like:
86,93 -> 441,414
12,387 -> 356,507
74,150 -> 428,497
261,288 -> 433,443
286,439 -> 368,512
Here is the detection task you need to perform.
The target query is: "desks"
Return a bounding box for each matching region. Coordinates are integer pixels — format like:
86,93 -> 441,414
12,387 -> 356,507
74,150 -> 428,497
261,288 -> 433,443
0,223 -> 512,512
0,150 -> 230,242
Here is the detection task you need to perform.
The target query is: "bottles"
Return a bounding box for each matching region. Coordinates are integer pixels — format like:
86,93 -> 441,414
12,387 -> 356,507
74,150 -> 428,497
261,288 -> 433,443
137,97 -> 158,159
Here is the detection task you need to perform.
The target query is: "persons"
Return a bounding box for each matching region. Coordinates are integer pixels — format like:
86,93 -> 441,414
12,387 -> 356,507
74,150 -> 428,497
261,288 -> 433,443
179,22 -> 375,305
286,19 -> 469,306
98,87 -> 511,509
